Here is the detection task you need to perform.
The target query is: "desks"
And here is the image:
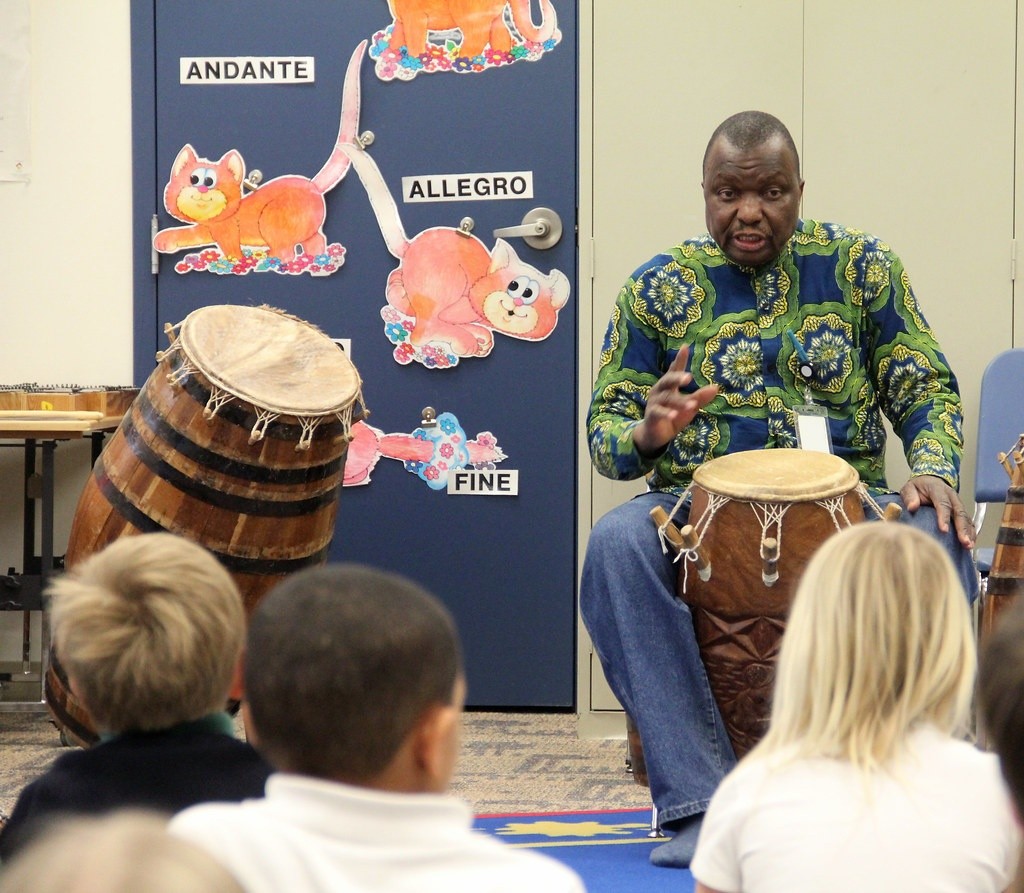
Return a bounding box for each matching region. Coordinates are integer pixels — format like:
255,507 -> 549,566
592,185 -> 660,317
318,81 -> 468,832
0,415 -> 130,745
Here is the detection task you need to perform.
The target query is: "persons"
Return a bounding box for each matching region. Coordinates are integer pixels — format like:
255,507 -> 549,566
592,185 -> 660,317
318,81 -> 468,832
0,531 -> 584,893
689,521 -> 1024,893
579,111 -> 980,867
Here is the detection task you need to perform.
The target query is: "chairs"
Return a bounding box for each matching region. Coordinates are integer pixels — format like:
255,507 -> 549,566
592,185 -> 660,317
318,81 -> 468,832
968,348 -> 1024,572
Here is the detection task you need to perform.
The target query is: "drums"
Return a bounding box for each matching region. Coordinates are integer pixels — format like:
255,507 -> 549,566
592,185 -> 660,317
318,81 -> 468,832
973,436 -> 1023,755
625,447 -> 866,786
45,303 -> 365,746
